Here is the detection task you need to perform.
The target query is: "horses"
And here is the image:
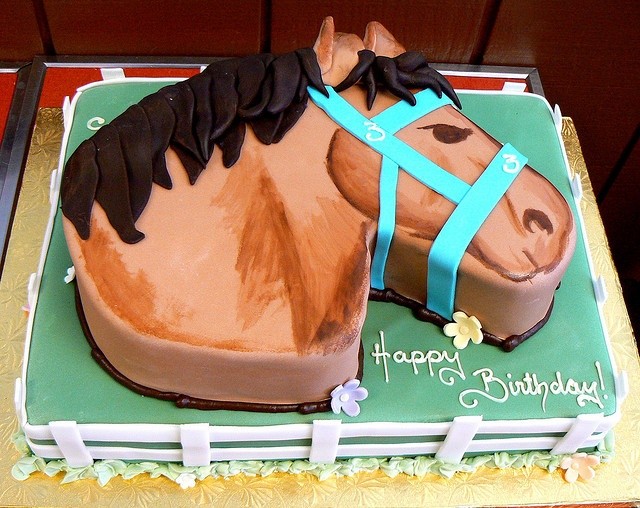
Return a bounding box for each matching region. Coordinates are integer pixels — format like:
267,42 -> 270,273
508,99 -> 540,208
56,14 -> 581,416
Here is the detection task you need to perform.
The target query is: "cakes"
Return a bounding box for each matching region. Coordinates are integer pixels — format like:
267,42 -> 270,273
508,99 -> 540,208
11,14 -> 630,471
